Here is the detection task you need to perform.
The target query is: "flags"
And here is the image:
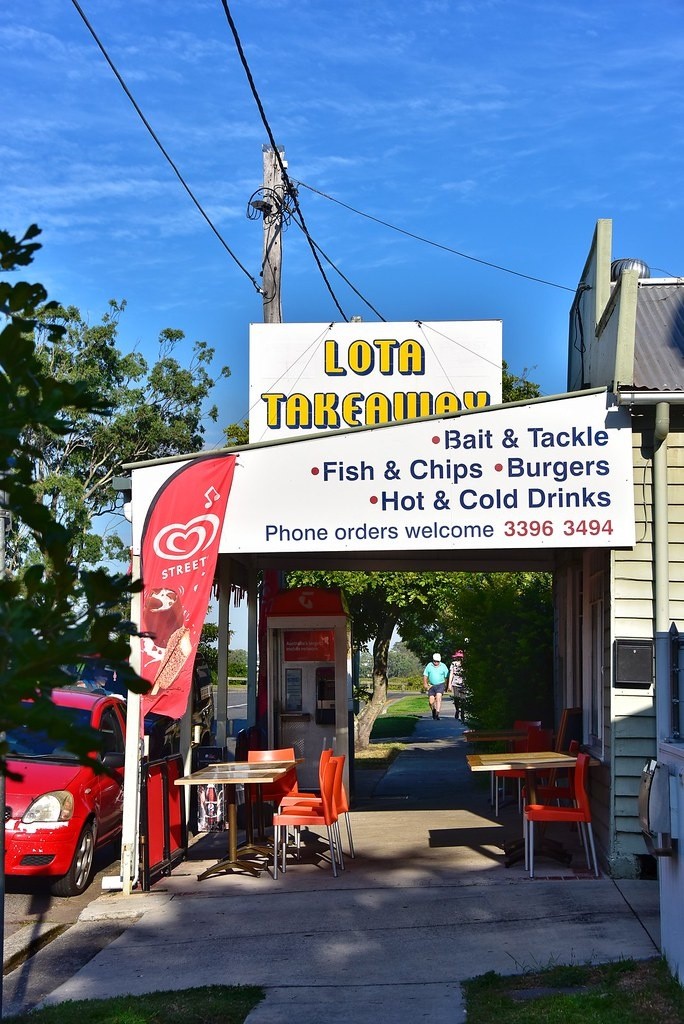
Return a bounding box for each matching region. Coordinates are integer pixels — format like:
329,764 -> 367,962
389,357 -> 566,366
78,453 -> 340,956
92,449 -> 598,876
139,455 -> 236,739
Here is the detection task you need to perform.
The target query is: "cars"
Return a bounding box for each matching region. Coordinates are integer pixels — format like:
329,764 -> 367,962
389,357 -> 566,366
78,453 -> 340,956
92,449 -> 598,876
0,689 -> 128,898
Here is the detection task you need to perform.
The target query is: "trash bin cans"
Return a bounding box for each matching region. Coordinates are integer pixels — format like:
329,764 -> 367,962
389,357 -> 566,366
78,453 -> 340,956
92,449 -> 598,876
197,745 -> 228,830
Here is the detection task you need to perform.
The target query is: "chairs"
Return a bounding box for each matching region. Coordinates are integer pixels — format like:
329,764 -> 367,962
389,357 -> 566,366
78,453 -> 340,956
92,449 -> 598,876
272,748 -> 359,879
498,720 -> 544,802
494,725 -> 555,817
522,736 -> 584,840
522,756 -> 607,878
248,746 -> 299,844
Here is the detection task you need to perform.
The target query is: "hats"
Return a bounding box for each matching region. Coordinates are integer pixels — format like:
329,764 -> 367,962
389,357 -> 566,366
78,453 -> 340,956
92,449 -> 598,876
452,650 -> 463,657
433,653 -> 441,661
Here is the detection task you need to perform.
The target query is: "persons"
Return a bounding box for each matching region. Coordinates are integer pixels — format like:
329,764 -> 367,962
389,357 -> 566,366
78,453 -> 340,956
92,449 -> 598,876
448,650 -> 469,724
423,653 -> 449,720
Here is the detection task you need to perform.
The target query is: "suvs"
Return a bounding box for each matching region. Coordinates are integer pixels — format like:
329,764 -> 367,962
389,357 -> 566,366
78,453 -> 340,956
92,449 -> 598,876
141,648 -> 215,750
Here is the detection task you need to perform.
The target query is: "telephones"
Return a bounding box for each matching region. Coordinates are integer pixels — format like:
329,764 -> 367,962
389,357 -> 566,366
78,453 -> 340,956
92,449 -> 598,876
316,667 -> 335,725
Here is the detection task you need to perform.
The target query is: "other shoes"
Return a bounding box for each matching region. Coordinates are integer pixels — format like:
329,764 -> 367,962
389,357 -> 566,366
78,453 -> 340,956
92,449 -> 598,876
455,710 -> 459,719
432,709 -> 436,720
436,715 -> 440,720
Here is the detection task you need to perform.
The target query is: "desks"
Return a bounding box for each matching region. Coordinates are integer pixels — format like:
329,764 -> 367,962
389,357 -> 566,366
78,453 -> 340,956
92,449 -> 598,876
208,756 -> 308,851
198,760 -> 299,864
463,726 -> 558,807
172,767 -> 286,882
465,746 -> 562,849
466,749 -> 581,873
